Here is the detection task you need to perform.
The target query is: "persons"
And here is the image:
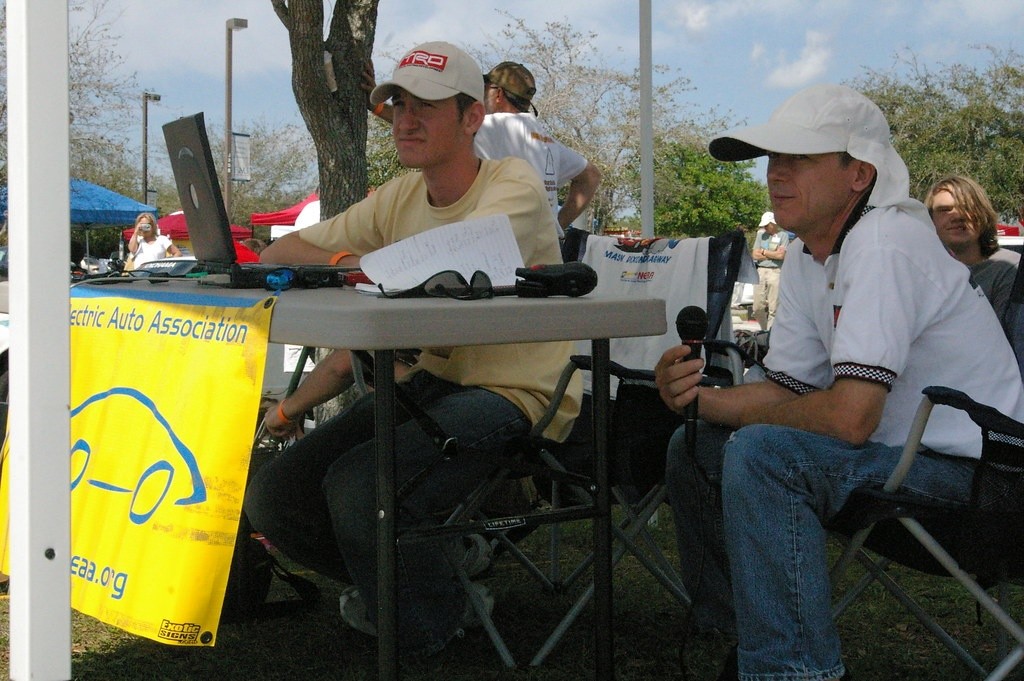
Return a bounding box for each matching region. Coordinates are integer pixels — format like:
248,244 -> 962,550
295,183 -> 321,231
924,176 -> 1024,384
128,213 -> 182,270
244,238 -> 267,255
237,42 -> 584,656
473,61 -> 602,238
653,84 -> 1024,681
751,211 -> 789,332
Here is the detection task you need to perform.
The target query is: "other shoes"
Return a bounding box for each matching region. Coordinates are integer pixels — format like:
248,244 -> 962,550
339,583 -> 496,638
464,532 -> 494,572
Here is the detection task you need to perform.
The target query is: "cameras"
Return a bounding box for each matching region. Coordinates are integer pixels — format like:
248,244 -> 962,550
140,224 -> 151,231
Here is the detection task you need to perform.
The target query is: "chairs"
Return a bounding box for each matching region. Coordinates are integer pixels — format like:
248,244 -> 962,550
281,228 -> 1024,681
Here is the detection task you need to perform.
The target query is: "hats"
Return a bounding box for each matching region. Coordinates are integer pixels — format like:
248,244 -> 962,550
480,61 -> 538,116
759,211 -> 776,226
369,40 -> 484,103
707,84 -> 910,205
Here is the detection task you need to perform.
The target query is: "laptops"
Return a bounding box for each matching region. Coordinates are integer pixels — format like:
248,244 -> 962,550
162,112 -> 361,287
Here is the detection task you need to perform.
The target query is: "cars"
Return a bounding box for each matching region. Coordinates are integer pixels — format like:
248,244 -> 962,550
0,246 -> 128,284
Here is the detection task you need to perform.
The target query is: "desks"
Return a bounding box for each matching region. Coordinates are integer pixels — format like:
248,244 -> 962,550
0,276 -> 668,681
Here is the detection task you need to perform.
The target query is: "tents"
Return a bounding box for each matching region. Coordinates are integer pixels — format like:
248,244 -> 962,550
123,208 -> 253,242
0,176 -> 158,275
250,191 -> 374,239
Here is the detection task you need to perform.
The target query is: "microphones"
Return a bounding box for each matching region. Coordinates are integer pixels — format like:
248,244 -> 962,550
676,305 -> 708,455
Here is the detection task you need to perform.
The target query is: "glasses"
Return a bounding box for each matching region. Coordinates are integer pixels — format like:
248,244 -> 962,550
263,265 -> 322,290
378,268 -> 493,301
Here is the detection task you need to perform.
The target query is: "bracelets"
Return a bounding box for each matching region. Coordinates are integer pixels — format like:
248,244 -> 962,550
329,251 -> 353,265
762,249 -> 764,254
277,400 -> 290,424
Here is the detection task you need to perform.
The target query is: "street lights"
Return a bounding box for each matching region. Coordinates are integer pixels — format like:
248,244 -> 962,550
142,91 -> 160,205
224,18 -> 248,225
